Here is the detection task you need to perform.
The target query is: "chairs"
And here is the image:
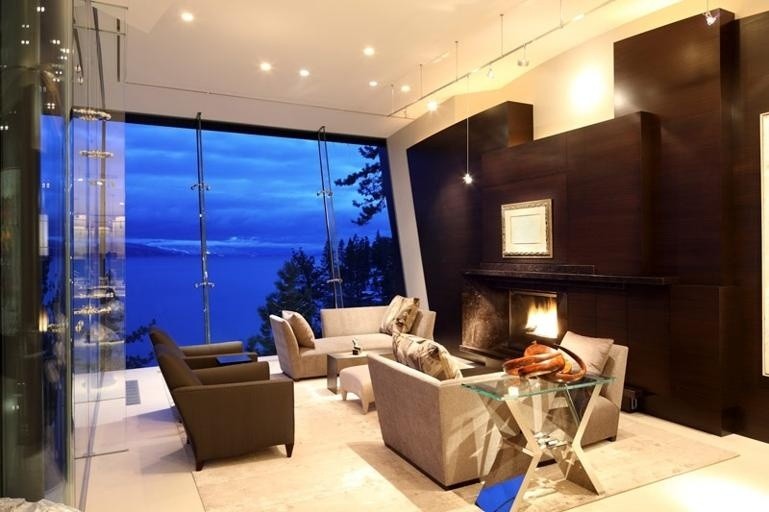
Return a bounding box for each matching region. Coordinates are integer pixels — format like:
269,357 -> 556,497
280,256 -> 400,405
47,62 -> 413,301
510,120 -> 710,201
147,339 -> 297,470
147,324 -> 261,372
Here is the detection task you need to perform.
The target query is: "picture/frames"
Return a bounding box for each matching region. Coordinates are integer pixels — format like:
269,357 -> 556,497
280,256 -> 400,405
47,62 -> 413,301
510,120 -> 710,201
497,199 -> 556,262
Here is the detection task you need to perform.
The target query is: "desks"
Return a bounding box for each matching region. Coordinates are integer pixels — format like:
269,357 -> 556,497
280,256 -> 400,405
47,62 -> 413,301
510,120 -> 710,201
462,367 -> 615,512
216,353 -> 252,366
324,348 -> 396,392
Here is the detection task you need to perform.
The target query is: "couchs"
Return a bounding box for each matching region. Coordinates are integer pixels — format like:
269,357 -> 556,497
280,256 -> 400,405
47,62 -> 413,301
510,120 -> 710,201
364,326 -> 631,492
265,297 -> 438,382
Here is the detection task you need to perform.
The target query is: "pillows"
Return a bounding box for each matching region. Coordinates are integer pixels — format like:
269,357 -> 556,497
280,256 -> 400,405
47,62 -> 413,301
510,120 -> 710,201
379,295 -> 420,337
281,309 -> 318,350
388,329 -> 465,381
557,331 -> 615,379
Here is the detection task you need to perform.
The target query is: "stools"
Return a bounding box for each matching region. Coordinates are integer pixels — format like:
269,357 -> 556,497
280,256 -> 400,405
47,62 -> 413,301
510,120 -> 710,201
335,363 -> 378,414
